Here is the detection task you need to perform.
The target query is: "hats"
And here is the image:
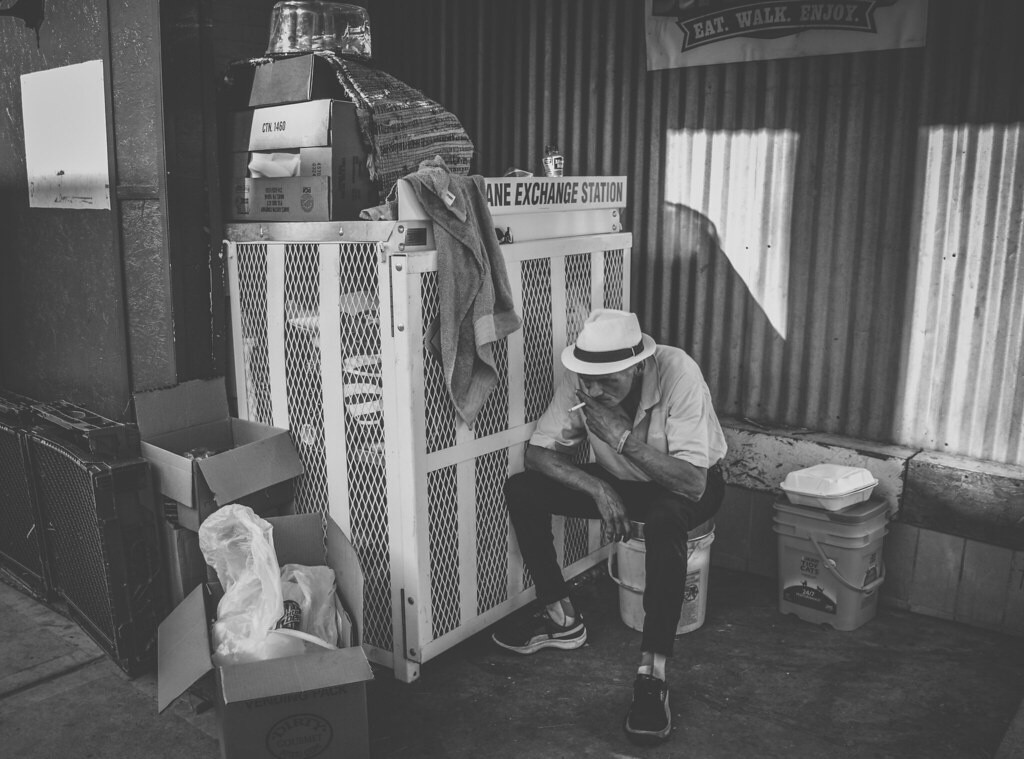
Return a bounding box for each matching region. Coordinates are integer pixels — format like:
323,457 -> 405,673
561,308 -> 657,374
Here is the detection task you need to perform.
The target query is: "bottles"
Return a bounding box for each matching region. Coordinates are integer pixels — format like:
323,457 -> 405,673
542,145 -> 564,177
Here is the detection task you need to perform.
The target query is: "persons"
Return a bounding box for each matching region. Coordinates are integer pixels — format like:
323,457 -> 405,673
492,309 -> 728,738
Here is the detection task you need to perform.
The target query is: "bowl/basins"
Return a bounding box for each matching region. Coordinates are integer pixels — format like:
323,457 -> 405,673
266,51 -> 373,99
264,1 -> 372,57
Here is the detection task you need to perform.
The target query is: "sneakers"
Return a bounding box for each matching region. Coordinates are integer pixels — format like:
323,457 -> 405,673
626,672 -> 672,737
492,607 -> 588,652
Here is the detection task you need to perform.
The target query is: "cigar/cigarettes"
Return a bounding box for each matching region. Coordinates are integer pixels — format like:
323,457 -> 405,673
568,402 -> 586,413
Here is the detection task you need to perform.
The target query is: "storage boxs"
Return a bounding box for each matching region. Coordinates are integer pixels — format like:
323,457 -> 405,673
132,376 -> 373,759
228,51 -> 377,222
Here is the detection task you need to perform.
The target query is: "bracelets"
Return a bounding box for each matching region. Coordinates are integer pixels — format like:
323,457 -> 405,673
618,429 -> 632,455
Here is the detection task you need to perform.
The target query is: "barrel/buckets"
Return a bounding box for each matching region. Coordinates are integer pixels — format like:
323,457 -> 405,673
608,520 -> 716,635
